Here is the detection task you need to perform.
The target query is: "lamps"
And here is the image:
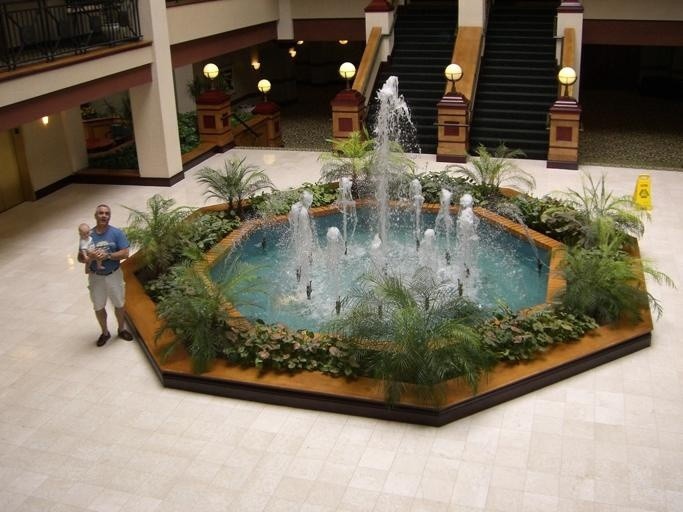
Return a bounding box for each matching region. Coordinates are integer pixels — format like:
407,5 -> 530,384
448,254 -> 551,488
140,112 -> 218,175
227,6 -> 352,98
555,65 -> 578,99
256,78 -> 273,102
443,63 -> 463,96
338,61 -> 358,92
202,62 -> 220,92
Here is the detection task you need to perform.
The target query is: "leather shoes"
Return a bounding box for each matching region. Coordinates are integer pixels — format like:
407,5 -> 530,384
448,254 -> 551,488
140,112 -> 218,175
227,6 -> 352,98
97,333 -> 110,346
118,328 -> 132,341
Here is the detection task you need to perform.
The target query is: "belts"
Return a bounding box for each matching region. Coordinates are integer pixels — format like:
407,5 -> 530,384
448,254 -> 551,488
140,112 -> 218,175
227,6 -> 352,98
90,265 -> 119,276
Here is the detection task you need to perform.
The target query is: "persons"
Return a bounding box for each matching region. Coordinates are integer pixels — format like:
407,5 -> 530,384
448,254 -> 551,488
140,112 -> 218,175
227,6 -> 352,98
77,204 -> 132,347
78,224 -> 106,274
